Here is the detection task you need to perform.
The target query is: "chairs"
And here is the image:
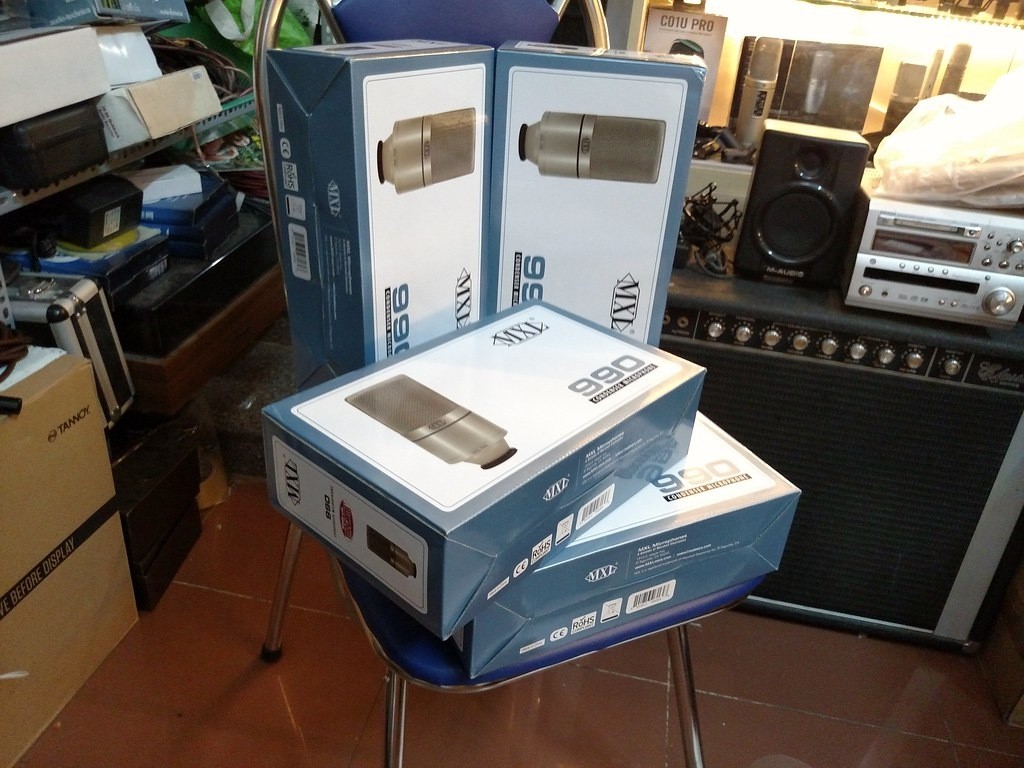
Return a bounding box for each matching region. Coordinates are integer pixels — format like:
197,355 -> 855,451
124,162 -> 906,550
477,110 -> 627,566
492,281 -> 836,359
248,0 -> 712,768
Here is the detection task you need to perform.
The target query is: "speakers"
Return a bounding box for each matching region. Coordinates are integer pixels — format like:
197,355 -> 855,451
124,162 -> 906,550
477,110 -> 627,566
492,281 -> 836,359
733,118 -> 871,291
658,223 -> 1024,655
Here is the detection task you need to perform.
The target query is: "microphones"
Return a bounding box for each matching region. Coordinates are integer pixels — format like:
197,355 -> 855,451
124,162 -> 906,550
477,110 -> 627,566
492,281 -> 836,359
882,43 -> 972,135
377,107 -> 476,194
345,374 -> 517,469
518,111 -> 666,183
803,51 -> 835,116
736,37 -> 784,148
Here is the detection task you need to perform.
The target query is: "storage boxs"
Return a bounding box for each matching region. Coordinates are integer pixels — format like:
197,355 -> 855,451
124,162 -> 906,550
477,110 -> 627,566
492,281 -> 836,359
641,0 -> 728,125
437,411 -> 801,679
259,301 -> 708,644
0,0 -> 241,768
262,35 -> 495,388
487,41 -> 706,344
725,36 -> 884,159
